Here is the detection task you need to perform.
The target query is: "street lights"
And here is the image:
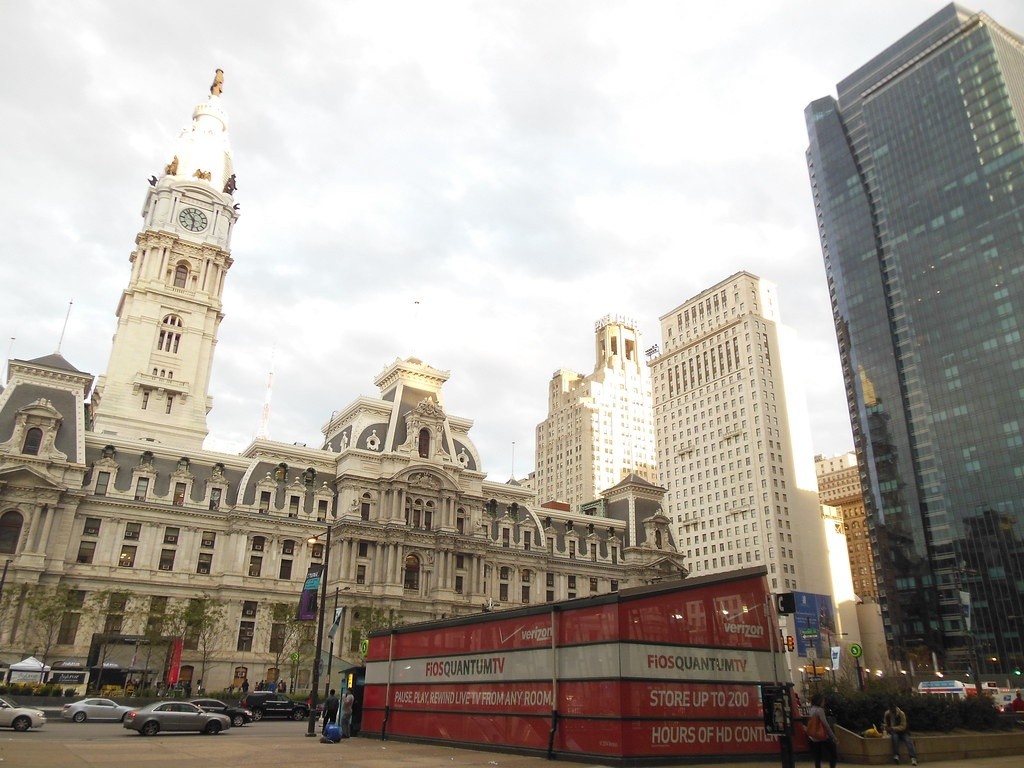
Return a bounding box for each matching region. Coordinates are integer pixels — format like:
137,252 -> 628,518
325,587 -> 350,697
308,529 -> 332,737
829,632 -> 848,693
952,567 -> 981,694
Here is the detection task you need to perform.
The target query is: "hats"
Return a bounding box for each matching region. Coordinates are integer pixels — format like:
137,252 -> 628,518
888,698 -> 896,705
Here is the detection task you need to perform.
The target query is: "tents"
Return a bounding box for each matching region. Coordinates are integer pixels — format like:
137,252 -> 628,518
4,655 -> 51,688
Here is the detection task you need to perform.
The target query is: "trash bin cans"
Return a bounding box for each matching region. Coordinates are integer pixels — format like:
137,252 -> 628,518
174,686 -> 184,697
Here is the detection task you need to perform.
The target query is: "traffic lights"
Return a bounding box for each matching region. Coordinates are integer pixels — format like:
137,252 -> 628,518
787,635 -> 795,652
1014,668 -> 1021,677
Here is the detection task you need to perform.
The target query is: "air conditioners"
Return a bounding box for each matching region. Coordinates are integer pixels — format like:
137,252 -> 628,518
251,570 -> 259,576
255,545 -> 262,550
238,672 -> 246,677
125,531 -> 133,536
204,540 -> 212,546
201,568 -> 207,574
163,565 -> 170,570
314,552 -> 321,557
168,536 -> 175,541
123,562 -> 131,567
358,579 -> 364,584
286,548 -> 292,553
88,529 -> 95,534
405,583 -> 411,589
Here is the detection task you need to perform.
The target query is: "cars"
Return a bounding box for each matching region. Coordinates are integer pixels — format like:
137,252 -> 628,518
0,697 -> 47,731
123,701 -> 231,735
189,698 -> 253,727
62,697 -> 135,723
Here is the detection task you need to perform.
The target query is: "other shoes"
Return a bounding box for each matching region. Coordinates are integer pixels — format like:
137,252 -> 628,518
911,758 -> 917,765
893,754 -> 899,761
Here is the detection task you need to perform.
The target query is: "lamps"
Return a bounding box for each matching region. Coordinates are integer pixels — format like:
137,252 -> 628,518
246,610 -> 254,615
247,631 -> 253,637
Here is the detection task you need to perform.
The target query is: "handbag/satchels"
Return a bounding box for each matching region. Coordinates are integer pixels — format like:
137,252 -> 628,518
805,707 -> 829,742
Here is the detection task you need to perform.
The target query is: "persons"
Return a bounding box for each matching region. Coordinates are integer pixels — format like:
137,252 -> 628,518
321,689 -> 339,735
806,694 -> 839,768
241,678 -> 286,694
308,691 -> 312,710
884,700 -> 918,766
340,688 -> 354,738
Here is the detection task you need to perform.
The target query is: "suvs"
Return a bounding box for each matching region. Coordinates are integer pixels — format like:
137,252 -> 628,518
240,690 -> 310,722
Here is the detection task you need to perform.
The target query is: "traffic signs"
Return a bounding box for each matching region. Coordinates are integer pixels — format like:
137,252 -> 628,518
802,634 -> 819,640
808,677 -> 822,682
